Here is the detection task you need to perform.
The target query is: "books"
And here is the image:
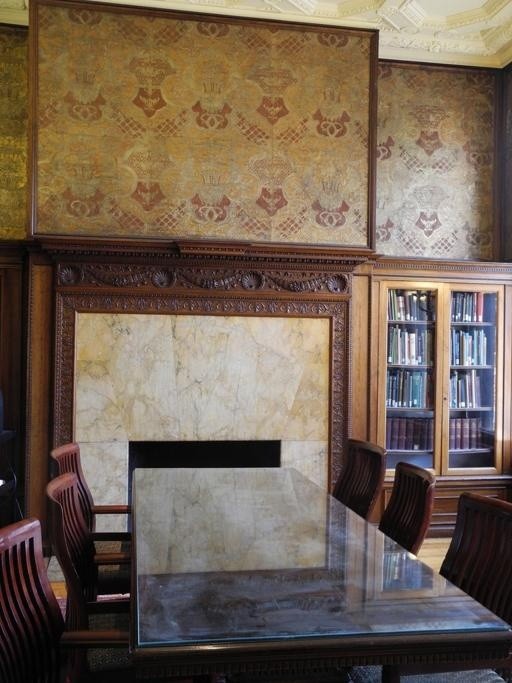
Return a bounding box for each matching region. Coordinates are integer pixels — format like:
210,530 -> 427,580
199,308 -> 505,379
387,288 -> 488,452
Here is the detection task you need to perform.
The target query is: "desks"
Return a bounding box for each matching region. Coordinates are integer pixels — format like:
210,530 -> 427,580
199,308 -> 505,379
127,467 -> 512,683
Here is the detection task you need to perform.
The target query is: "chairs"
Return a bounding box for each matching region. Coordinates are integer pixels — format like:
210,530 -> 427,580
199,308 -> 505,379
332,437 -> 388,520
377,459 -> 436,557
381,488 -> 512,681
45,471 -> 128,628
50,443 -> 138,591
2,514 -> 137,683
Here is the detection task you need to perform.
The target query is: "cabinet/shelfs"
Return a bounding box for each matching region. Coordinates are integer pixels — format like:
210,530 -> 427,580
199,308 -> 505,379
370,274 -> 505,483
